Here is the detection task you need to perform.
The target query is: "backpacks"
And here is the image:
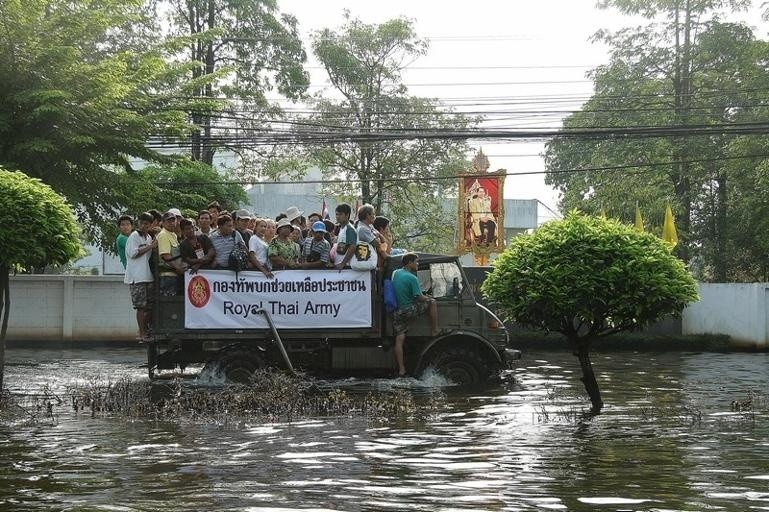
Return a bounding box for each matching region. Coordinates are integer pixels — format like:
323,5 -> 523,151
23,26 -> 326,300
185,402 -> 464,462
229,230 -> 248,270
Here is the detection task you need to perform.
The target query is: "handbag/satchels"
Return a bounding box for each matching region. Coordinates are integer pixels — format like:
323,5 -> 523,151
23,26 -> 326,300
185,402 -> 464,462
383,279 -> 397,313
350,225 -> 377,270
307,250 -> 321,262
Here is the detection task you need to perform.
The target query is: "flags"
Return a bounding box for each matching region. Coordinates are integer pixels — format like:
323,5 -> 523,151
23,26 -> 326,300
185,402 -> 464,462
635,199 -> 645,231
320,197 -> 330,220
662,201 -> 680,250
598,201 -> 607,217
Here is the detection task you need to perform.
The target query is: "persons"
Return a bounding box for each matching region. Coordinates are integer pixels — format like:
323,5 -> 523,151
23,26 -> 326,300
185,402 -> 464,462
390,252 -> 443,378
467,187 -> 498,248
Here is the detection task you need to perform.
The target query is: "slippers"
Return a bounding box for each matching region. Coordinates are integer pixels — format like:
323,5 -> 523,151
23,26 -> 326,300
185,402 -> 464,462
433,327 -> 452,335
399,371 -> 413,378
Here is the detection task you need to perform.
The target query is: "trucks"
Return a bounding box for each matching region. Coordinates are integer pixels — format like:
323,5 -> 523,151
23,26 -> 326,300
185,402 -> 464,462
130,239 -> 521,388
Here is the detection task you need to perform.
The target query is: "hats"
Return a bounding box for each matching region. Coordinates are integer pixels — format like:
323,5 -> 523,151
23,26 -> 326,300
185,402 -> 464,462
285,206 -> 305,219
162,207 -> 183,222
276,218 -> 294,234
236,208 -> 259,221
312,221 -> 328,232
